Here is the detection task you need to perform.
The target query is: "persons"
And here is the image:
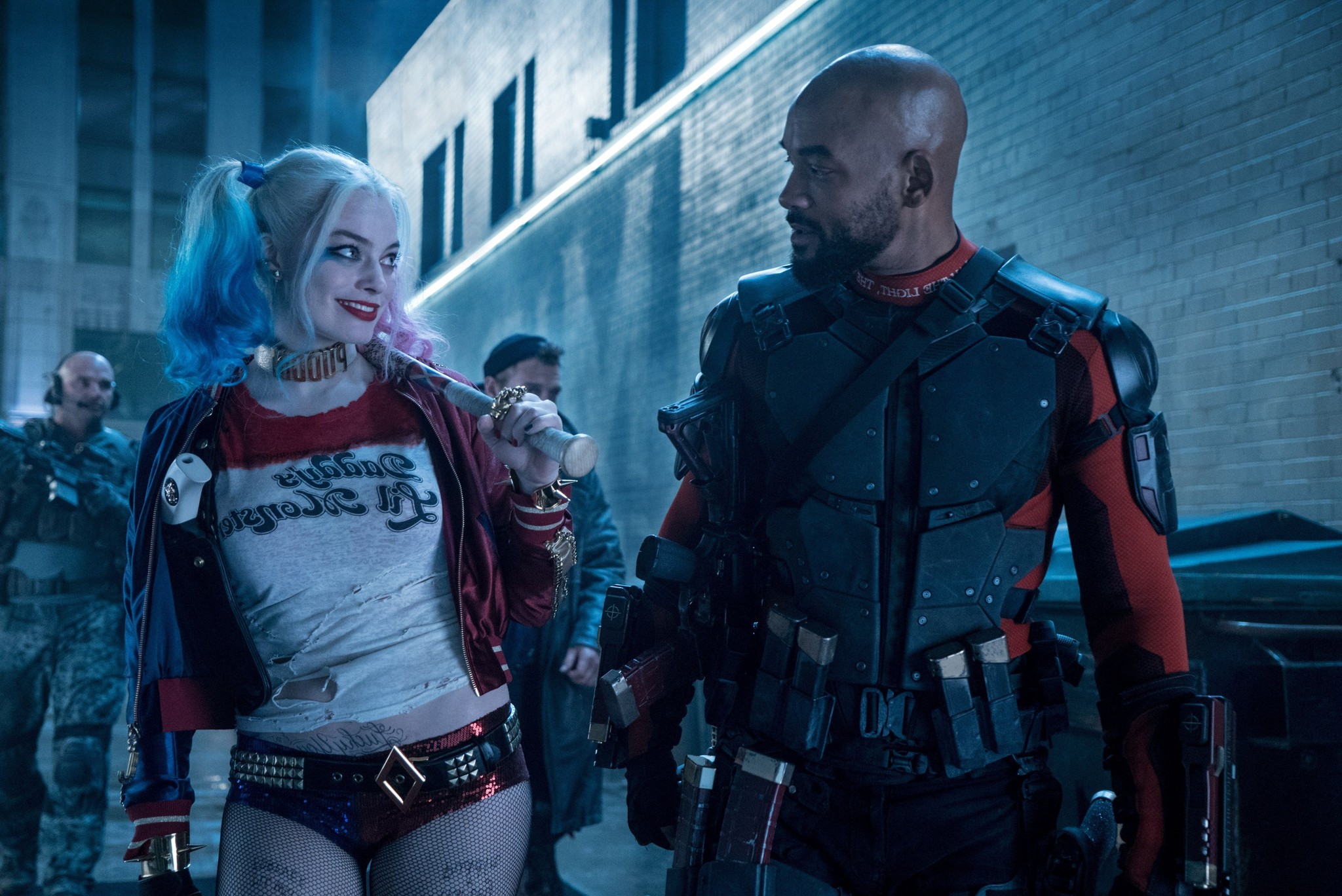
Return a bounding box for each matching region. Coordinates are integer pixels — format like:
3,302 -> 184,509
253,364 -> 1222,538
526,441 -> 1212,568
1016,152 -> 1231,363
587,44 -> 1237,896
118,148 -> 600,896
476,332 -> 627,896
0,351 -> 141,896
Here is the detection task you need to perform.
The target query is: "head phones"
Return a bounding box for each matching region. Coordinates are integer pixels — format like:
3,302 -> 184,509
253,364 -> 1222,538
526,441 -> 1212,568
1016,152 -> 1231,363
45,353 -> 120,411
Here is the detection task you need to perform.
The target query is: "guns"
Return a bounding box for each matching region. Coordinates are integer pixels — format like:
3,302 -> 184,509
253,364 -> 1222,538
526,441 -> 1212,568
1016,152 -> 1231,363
636,384 -> 765,727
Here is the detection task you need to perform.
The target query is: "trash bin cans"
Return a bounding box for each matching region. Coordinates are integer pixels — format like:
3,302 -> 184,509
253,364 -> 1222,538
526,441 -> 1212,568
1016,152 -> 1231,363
1036,502 -> 1340,896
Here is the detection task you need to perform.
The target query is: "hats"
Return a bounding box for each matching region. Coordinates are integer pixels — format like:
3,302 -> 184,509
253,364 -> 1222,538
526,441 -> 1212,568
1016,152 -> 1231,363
484,333 -> 545,377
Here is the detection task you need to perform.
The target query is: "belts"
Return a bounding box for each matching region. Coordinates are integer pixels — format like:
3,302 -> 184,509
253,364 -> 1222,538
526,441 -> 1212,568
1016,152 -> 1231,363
230,706 -> 522,805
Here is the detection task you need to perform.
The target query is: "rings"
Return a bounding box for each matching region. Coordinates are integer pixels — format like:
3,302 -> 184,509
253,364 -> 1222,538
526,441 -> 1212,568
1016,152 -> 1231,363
490,385 -> 527,420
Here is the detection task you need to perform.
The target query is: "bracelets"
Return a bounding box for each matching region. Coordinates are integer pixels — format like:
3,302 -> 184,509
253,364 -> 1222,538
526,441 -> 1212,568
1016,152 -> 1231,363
495,464 -> 578,512
123,831 -> 208,881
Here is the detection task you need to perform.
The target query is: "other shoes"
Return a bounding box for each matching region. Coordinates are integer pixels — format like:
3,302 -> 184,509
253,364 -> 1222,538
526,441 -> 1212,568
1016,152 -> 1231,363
520,851 -> 580,896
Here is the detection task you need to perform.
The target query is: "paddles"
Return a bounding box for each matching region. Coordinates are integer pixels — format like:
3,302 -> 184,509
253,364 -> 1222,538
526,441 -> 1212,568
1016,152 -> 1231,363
158,453 -> 269,715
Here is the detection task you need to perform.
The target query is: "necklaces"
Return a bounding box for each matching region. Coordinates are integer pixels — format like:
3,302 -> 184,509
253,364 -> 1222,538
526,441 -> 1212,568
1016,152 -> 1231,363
254,343 -> 357,382
